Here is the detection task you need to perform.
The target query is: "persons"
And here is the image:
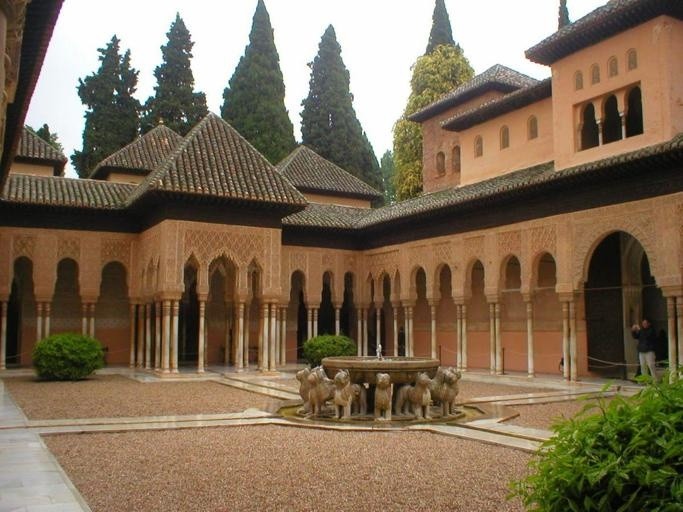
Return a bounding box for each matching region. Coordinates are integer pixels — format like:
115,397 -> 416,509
631,318 -> 657,381
